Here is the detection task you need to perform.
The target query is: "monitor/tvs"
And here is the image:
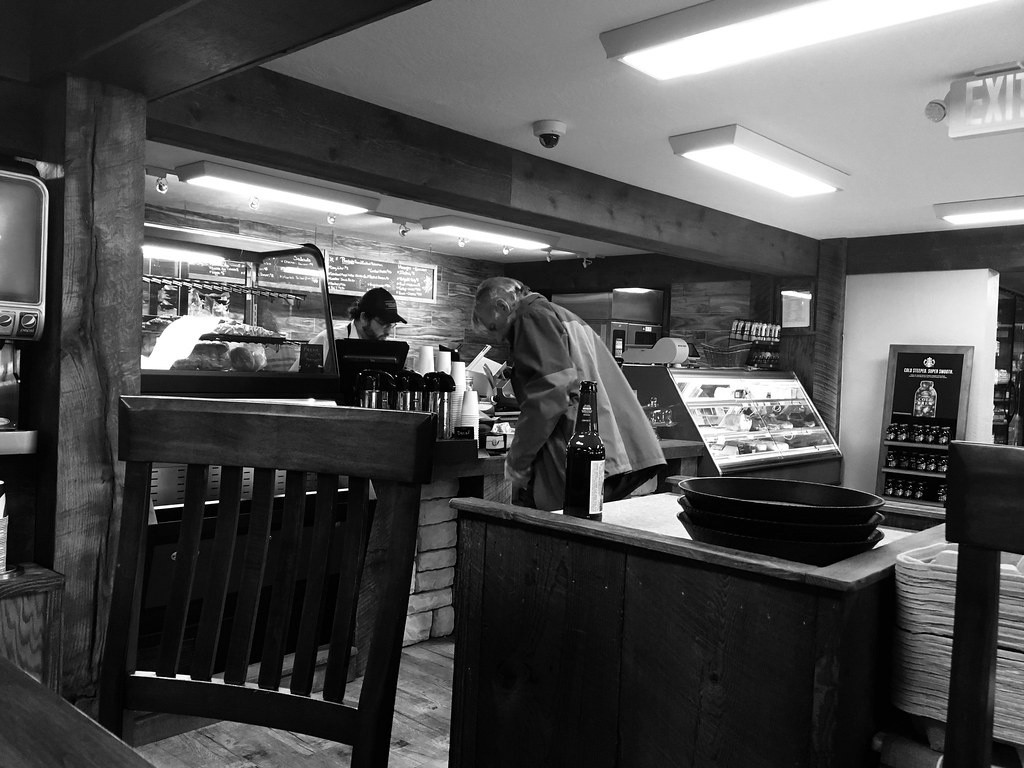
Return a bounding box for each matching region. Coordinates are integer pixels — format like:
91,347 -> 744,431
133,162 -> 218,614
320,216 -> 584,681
324,337 -> 409,381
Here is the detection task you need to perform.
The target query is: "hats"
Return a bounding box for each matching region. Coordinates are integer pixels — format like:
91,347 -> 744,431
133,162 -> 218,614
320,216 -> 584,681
358,287 -> 407,324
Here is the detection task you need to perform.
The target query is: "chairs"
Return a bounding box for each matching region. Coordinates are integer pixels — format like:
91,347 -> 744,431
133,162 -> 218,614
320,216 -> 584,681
96,394 -> 439,768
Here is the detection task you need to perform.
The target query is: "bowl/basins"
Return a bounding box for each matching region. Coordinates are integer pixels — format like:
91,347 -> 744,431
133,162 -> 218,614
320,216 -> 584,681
676,479 -> 885,558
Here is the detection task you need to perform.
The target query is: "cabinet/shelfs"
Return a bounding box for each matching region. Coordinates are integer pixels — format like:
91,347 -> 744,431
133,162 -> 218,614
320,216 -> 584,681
874,414 -> 957,521
140,234 -> 342,378
667,368 -> 840,476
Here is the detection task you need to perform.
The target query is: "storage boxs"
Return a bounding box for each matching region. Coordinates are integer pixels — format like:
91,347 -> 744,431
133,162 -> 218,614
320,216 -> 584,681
676,479 -> 885,568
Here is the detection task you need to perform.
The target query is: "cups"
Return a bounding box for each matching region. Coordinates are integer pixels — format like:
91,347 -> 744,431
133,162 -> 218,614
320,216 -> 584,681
434,351 -> 451,374
450,362 -> 465,433
461,392 -> 479,449
413,346 -> 434,376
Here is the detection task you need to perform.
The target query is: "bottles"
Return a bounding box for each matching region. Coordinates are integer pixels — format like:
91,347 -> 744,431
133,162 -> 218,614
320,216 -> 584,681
885,476 -> 947,503
886,450 -> 948,474
563,381 -> 606,522
913,380 -> 937,417
886,423 -> 951,446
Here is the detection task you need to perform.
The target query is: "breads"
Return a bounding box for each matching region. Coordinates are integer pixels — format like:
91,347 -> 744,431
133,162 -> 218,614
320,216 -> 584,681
143,316 -> 281,372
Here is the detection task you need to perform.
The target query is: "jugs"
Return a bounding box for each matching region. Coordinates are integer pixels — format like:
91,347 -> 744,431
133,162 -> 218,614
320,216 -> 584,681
424,371 -> 455,439
395,371 -> 423,411
357,370 -> 395,409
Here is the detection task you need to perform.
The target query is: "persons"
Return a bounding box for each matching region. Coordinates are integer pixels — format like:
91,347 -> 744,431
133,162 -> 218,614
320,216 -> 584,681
470,276 -> 667,512
288,287 -> 407,372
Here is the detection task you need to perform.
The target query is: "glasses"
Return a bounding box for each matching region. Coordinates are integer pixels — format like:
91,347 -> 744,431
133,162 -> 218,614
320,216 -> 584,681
374,317 -> 397,329
